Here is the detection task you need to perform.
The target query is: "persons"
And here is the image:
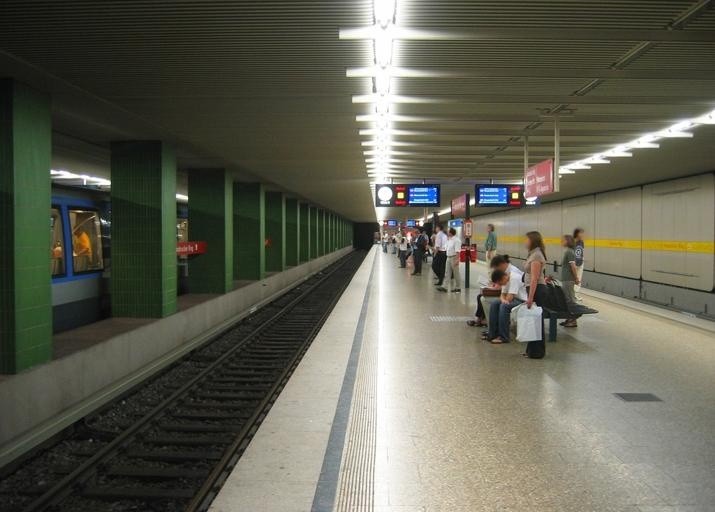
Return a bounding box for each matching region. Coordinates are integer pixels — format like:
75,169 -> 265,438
559,235 -> 579,328
484,223 -> 496,264
436,228 -> 461,292
465,254 -> 524,327
380,224 -> 449,288
70,225 -> 92,264
572,227 -> 585,301
522,229 -> 548,359
481,269 -> 527,344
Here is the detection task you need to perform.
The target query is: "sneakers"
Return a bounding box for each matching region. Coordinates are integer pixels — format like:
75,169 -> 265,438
559,319 -> 577,327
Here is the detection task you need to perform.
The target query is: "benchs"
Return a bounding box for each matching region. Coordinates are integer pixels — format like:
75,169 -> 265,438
543,302 -> 599,342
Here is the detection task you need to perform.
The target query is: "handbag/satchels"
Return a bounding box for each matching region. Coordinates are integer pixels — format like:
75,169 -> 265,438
510,301 -> 544,343
534,276 -> 570,320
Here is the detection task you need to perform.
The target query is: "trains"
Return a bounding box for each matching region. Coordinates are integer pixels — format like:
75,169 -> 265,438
51,193 -> 189,334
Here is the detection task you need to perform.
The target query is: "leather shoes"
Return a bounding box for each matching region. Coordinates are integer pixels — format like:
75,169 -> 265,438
436,286 -> 461,292
466,317 -> 509,344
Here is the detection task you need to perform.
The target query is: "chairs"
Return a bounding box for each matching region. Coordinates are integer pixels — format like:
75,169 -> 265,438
50,254 -> 88,275
